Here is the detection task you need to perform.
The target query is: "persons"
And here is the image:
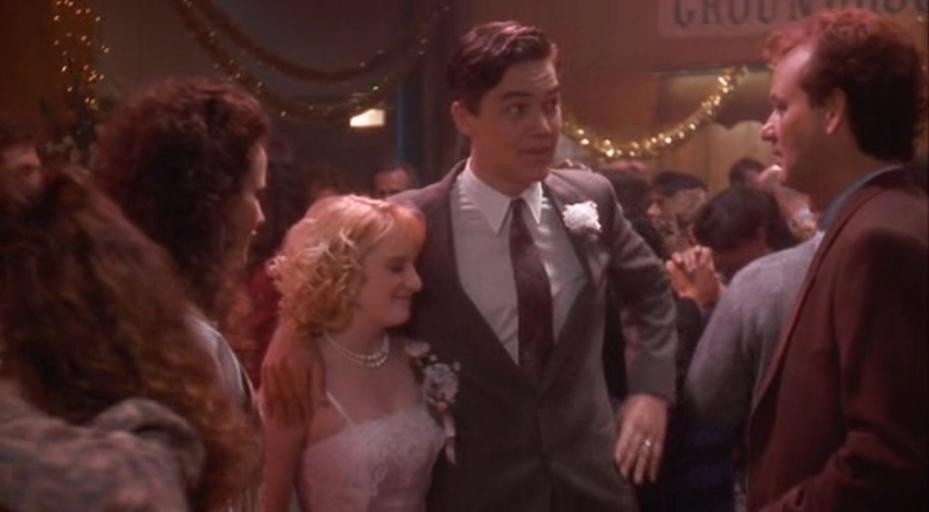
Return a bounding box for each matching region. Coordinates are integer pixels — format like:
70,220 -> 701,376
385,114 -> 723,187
742,10 -> 928,509
93,78 -> 271,511
259,153 -> 446,511
1,129 -> 267,511
385,20 -> 679,510
600,157 -> 825,510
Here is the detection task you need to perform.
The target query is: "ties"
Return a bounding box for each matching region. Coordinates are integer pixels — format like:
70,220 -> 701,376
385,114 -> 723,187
506,199 -> 554,394
